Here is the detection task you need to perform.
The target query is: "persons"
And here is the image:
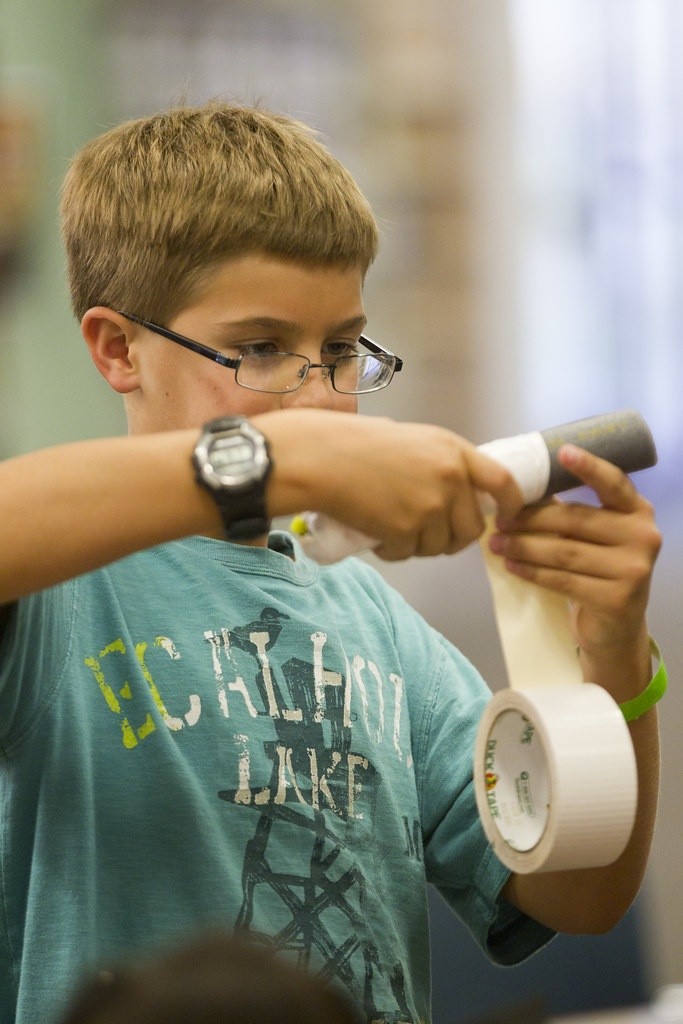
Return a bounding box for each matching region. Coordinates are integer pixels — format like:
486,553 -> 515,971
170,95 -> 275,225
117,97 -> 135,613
0,97 -> 669,1024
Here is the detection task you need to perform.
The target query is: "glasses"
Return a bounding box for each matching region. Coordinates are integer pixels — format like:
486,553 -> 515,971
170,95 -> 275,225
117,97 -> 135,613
115,310 -> 404,395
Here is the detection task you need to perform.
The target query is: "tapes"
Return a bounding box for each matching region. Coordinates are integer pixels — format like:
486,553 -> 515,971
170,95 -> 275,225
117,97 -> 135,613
472,681 -> 636,878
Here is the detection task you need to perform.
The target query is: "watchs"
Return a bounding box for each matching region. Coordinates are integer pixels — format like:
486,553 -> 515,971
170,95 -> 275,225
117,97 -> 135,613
193,414 -> 277,546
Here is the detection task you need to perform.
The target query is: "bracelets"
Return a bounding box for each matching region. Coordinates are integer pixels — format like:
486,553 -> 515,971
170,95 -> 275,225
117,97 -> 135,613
565,633 -> 664,726
